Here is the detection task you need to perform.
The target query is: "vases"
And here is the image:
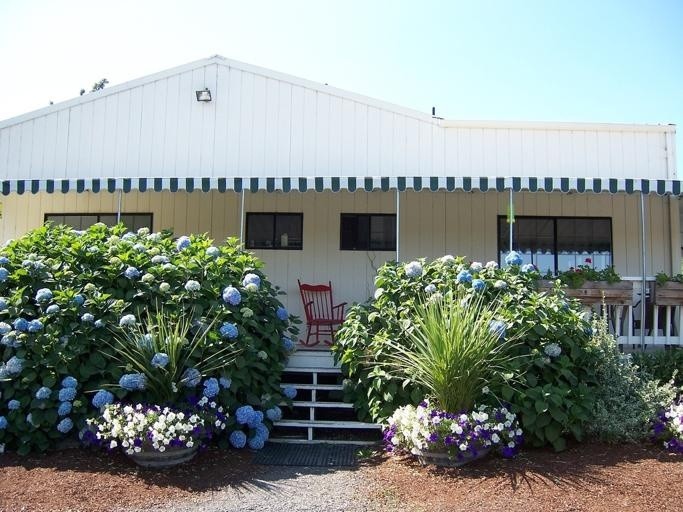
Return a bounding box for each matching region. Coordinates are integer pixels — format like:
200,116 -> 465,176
533,279 -> 634,307
132,447 -> 199,468
408,447 -> 489,467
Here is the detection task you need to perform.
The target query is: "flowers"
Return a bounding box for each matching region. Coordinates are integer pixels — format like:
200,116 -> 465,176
541,257 -> 622,292
359,282 -> 533,466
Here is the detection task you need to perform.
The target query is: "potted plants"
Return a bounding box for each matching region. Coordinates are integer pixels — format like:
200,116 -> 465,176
654,272 -> 683,306
81,293 -> 246,456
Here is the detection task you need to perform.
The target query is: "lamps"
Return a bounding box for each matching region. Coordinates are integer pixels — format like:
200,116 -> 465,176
196,87 -> 211,102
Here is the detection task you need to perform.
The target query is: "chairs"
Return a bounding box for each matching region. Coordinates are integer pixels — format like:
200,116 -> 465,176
297,277 -> 348,350
631,295 -> 679,337
